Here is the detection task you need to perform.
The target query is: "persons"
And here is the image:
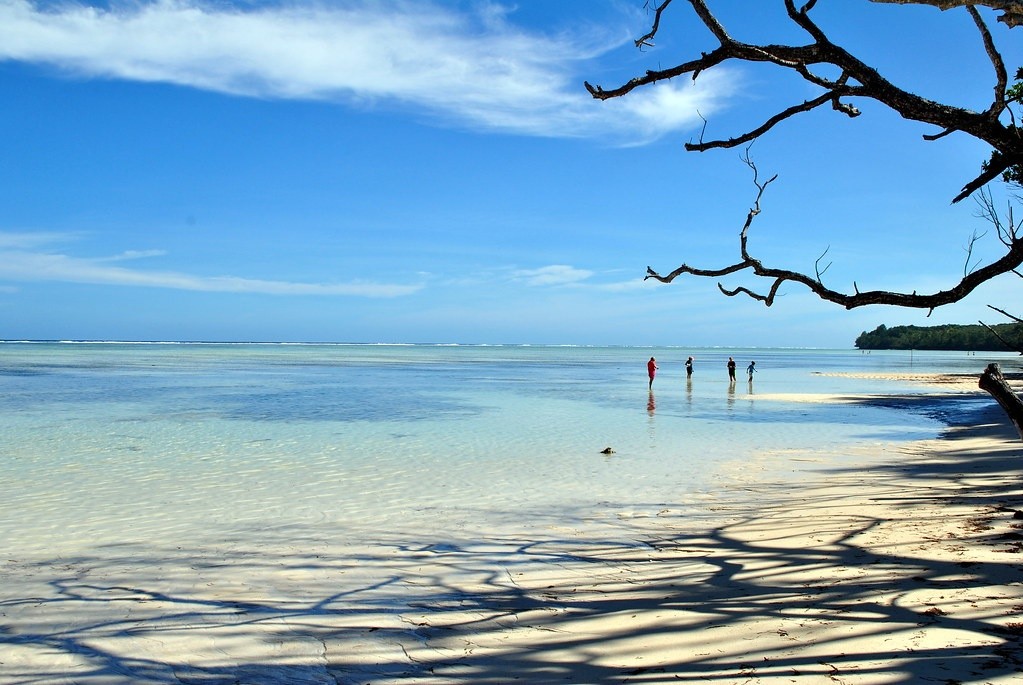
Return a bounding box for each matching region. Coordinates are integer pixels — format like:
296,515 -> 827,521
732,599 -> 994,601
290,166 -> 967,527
647,357 -> 659,388
746,362 -> 756,382
684,357 -> 694,379
727,357 -> 737,381
